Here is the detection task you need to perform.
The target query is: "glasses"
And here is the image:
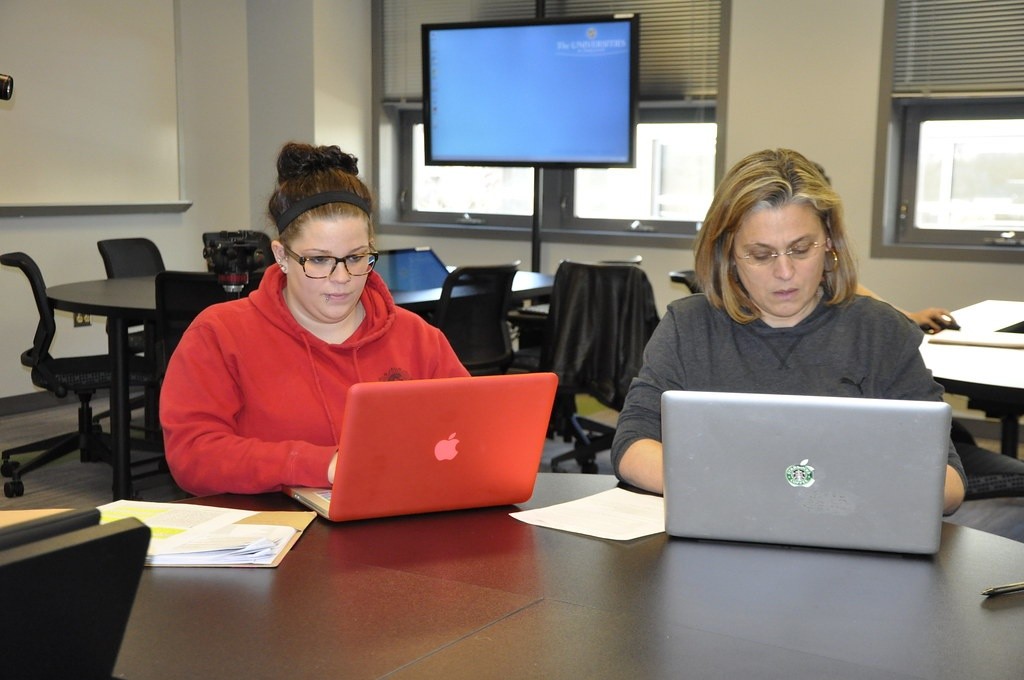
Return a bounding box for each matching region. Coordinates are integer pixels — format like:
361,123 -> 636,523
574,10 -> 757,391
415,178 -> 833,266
284,243 -> 380,278
733,224 -> 831,266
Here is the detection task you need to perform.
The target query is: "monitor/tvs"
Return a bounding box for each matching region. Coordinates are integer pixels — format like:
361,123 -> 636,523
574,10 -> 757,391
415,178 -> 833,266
421,12 -> 640,168
370,247 -> 451,294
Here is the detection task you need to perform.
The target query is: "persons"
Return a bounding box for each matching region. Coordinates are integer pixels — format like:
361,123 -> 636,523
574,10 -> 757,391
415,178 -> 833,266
852,284 -> 956,334
159,141 -> 472,498
610,147 -> 968,518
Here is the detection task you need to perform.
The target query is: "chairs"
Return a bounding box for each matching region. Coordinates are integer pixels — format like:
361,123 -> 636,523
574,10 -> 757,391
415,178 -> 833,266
430,255 -> 700,474
1,232 -> 275,499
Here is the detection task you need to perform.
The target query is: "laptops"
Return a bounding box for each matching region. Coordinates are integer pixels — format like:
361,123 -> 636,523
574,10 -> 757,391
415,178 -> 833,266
1,515 -> 152,680
662,389 -> 952,557
283,371 -> 558,522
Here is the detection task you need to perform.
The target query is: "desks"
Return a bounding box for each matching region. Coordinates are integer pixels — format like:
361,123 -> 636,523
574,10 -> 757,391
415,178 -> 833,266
112,473 -> 1023,679
918,300 -> 1024,460
45,266 -> 553,500
